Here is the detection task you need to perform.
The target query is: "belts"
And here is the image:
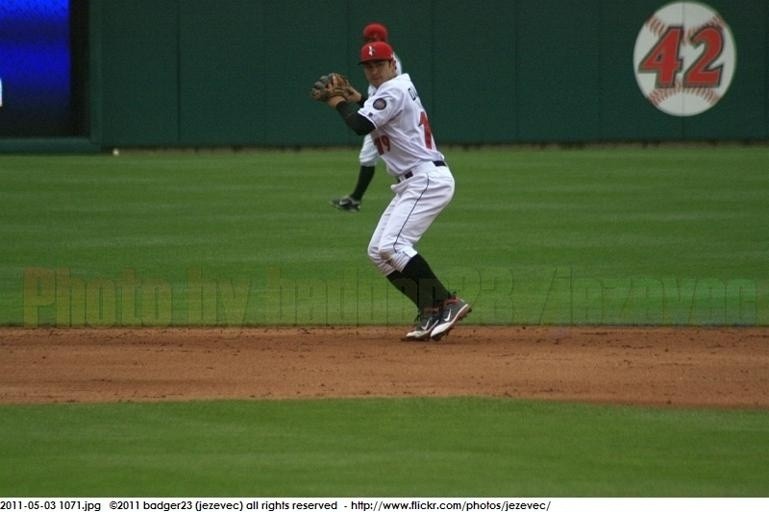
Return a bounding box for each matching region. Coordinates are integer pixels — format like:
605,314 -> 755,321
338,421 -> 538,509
396,160 -> 445,183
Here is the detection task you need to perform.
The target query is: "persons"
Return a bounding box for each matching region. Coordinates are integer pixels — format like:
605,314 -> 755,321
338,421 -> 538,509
328,22 -> 403,214
310,41 -> 472,341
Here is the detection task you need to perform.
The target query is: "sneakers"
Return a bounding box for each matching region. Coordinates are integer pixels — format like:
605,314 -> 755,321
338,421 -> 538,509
405,306 -> 441,341
327,195 -> 361,213
430,293 -> 472,341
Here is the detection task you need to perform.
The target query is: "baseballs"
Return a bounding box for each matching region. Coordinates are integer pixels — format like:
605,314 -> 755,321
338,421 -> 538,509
113,150 -> 119,156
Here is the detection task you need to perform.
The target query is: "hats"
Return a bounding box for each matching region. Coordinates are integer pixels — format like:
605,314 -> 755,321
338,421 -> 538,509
358,41 -> 392,64
363,24 -> 387,42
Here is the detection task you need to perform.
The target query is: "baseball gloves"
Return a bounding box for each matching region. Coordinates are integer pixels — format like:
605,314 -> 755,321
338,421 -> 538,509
310,71 -> 350,103
